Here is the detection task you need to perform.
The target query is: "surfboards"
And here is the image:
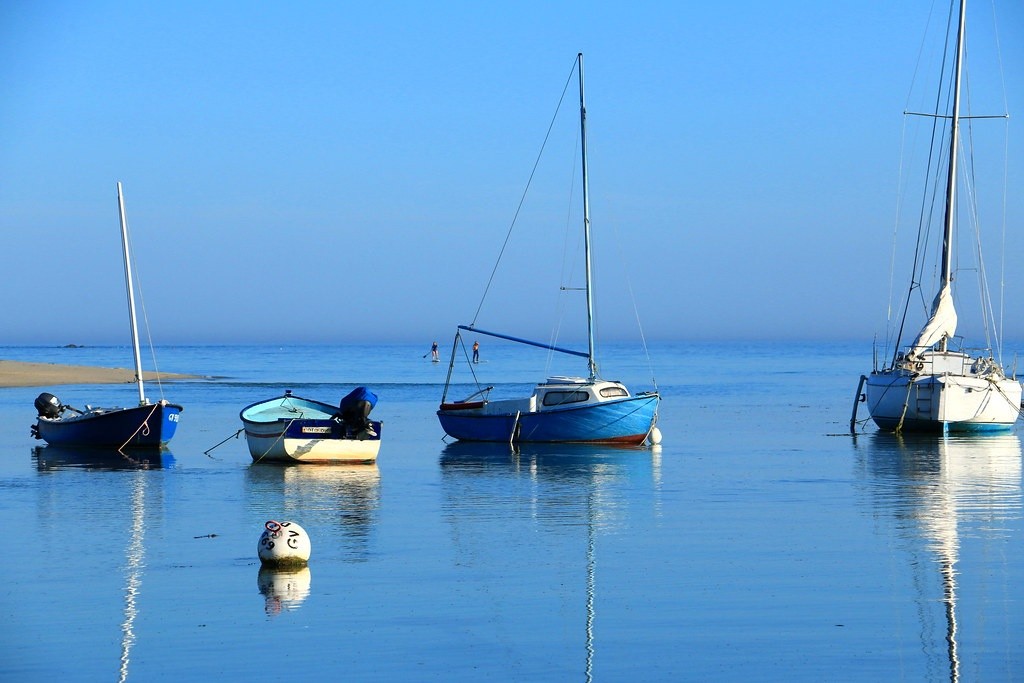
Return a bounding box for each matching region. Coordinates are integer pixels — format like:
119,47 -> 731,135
465,360 -> 488,363
433,360 -> 440,362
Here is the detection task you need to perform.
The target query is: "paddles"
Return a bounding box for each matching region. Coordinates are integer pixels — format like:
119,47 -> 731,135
423,350 -> 432,358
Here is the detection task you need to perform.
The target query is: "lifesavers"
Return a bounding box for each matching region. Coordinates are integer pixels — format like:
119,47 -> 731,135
971,359 -> 987,374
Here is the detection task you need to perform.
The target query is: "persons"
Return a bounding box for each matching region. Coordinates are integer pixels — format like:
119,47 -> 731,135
431,341 -> 439,361
472,340 -> 480,363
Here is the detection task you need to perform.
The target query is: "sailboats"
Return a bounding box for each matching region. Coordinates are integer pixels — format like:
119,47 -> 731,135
29,443 -> 176,597
865,420 -> 1022,683
435,52 -> 663,447
847,0 -> 1023,439
438,436 -> 653,683
30,180 -> 182,447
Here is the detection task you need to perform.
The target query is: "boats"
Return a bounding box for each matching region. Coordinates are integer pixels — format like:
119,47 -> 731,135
240,386 -> 386,464
249,457 -> 381,491
